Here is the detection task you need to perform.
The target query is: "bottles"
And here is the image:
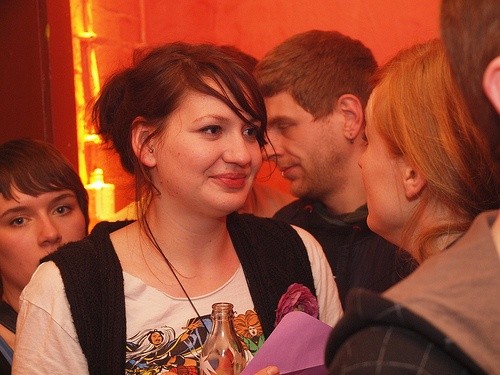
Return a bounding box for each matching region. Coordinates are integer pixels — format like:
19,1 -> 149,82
200,303 -> 246,375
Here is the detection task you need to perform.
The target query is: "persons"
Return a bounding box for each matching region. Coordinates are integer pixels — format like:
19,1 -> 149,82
9,39 -> 345,375
325,0 -> 500,375
250,28 -> 416,298
362,39 -> 492,268
0,136 -> 89,374
